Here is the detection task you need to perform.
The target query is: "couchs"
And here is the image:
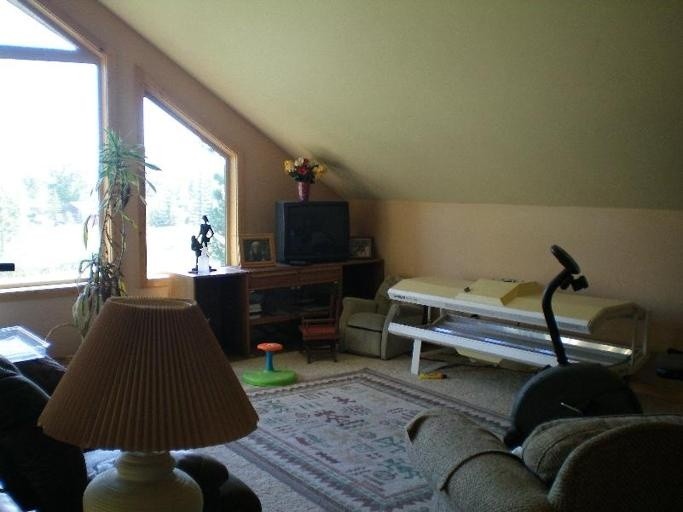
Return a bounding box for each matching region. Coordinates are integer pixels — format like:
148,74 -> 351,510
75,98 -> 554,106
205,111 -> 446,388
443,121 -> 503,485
0,354 -> 262,512
339,273 -> 424,360
403,405 -> 683,512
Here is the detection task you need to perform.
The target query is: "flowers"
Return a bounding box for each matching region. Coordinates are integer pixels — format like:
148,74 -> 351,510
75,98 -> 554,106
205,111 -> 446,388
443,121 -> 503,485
283,156 -> 326,184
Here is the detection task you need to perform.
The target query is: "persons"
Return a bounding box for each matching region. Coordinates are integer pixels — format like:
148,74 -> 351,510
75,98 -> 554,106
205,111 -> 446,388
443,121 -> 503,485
246,241 -> 268,261
191,235 -> 203,259
198,213 -> 214,248
351,240 -> 372,257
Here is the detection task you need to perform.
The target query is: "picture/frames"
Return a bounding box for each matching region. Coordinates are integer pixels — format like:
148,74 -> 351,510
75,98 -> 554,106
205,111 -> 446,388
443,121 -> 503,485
347,236 -> 375,259
239,232 -> 276,269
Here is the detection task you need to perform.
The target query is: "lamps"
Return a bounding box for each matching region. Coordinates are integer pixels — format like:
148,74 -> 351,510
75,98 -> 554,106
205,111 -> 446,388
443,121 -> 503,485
38,297 -> 258,512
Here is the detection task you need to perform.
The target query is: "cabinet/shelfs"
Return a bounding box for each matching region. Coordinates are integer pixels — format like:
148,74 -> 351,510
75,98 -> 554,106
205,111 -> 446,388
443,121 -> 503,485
194,258 -> 385,357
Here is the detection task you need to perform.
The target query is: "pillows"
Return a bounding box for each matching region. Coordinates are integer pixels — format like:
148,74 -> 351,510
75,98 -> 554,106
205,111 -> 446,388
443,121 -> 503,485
35,358 -> 68,396
522,414 -> 682,485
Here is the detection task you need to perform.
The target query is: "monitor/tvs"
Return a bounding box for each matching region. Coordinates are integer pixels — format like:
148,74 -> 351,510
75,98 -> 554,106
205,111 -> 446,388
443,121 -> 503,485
276,199 -> 352,266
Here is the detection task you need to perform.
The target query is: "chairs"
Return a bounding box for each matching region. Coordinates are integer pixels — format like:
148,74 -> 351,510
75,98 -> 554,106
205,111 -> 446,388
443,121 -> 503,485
298,280 -> 342,364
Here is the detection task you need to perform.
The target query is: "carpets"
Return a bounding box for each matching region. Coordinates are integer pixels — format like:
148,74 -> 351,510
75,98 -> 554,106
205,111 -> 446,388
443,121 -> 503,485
225,368 -> 510,512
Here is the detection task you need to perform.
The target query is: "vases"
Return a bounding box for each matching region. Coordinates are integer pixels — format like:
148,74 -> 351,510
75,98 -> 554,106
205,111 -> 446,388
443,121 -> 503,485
298,182 -> 310,201
